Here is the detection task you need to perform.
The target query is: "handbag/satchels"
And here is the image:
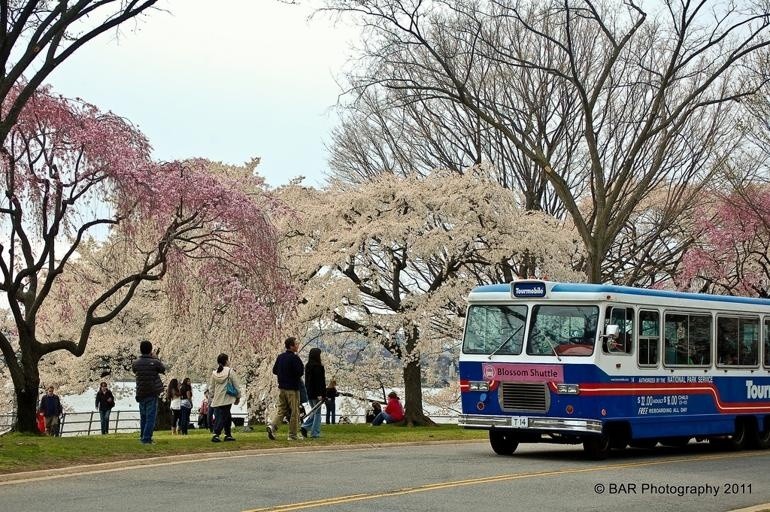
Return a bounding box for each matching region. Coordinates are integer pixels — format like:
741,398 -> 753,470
226,383 -> 238,396
181,399 -> 192,408
169,396 -> 181,410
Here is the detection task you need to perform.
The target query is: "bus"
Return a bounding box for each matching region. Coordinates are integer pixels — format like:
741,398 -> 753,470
455,274 -> 770,457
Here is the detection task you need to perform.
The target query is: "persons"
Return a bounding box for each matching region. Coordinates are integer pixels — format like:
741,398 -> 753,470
371,392 -> 404,425
95,382 -> 115,435
325,381 -> 338,424
266,337 -> 304,441
208,352 -> 241,442
167,377 -> 213,436
301,348 -> 326,438
40,387 -> 59,438
131,341 -> 165,443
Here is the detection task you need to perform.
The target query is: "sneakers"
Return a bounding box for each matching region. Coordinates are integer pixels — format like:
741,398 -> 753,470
212,436 -> 221,442
300,427 -> 307,438
266,425 -> 276,440
224,436 -> 235,441
288,436 -> 302,440
